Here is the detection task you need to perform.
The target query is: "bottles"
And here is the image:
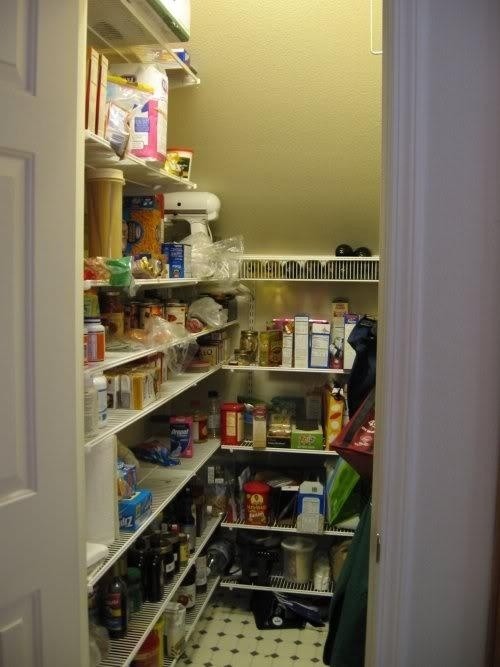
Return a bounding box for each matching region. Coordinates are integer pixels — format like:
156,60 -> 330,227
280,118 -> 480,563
86,166 -> 127,260
87,511 -> 164,643
178,466 -> 210,611
191,388 -> 221,443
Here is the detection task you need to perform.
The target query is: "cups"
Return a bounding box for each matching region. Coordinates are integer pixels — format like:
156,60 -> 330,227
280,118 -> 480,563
282,537 -> 317,583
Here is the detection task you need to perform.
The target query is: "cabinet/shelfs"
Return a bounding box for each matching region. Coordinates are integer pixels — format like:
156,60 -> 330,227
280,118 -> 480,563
220,255 -> 380,603
82,0 -> 224,666
